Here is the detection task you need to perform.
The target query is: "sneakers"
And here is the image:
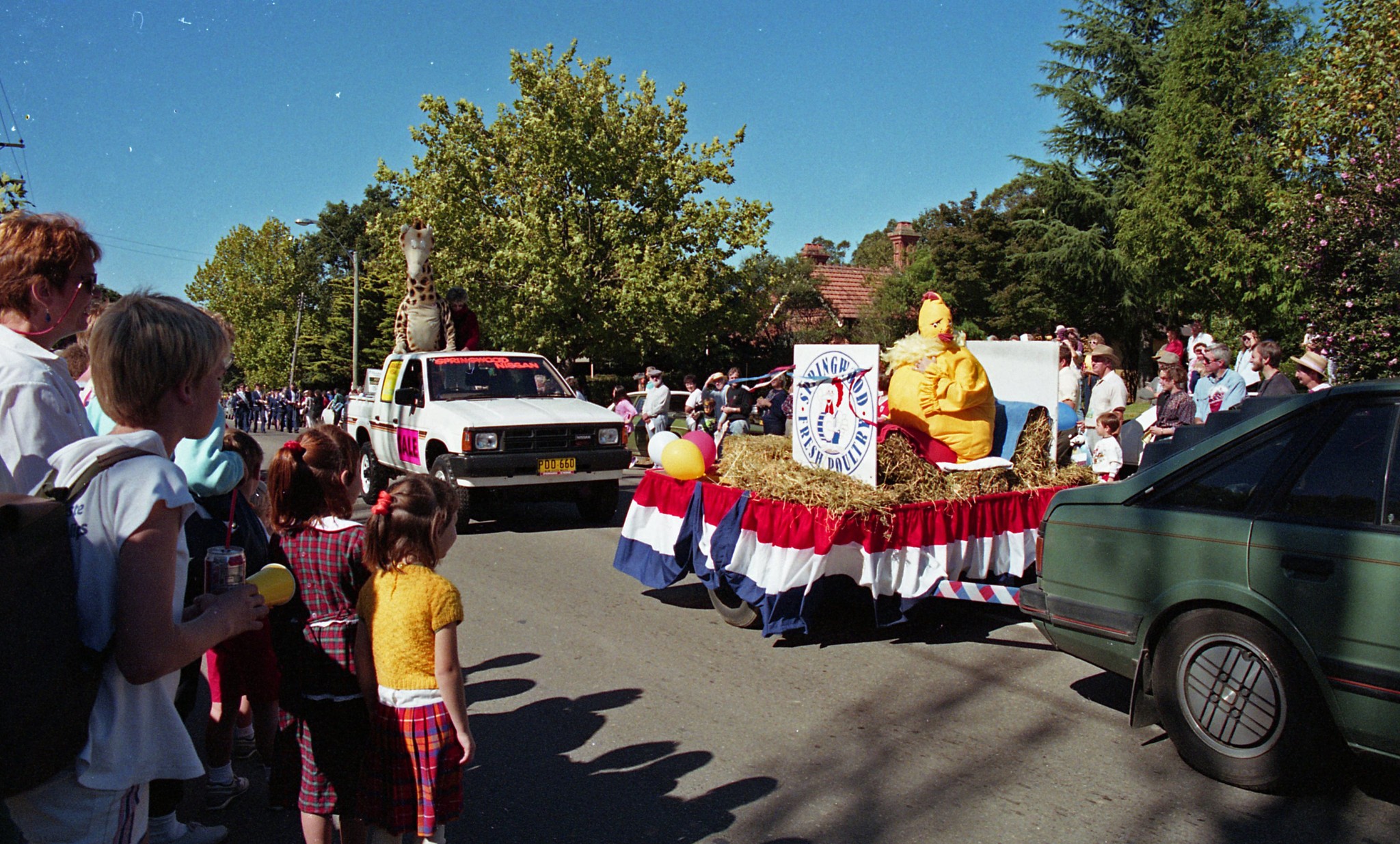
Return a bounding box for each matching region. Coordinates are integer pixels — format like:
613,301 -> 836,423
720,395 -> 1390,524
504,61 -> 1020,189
233,733 -> 260,760
203,773 -> 251,811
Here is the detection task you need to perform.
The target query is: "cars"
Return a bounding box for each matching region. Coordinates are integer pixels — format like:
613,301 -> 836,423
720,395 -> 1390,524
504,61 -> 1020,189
607,390 -> 764,467
1019,381 -> 1400,791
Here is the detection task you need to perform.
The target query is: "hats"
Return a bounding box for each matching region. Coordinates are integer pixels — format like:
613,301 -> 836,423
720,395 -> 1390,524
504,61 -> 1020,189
1157,352 -> 1180,366
706,371 -> 728,386
1289,350 -> 1328,379
1081,344 -> 1122,368
418,366 -> 447,383
1064,326 -> 1076,333
1306,323 -> 1316,329
445,287 -> 464,302
1054,324 -> 1066,333
648,369 -> 662,376
1151,349 -> 1169,358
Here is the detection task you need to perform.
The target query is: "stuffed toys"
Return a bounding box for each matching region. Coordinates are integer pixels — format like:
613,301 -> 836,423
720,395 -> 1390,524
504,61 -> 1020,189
879,291 -> 997,465
388,216 -> 458,356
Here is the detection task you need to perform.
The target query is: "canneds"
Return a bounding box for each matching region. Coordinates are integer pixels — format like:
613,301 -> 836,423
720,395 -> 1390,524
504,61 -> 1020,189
203,546 -> 246,595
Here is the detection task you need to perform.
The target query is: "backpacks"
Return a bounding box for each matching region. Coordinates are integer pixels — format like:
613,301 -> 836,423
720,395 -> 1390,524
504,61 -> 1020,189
0,442 -> 162,800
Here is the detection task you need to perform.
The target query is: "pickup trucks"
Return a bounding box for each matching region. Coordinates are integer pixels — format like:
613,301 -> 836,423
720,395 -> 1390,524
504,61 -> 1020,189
347,349 -> 631,534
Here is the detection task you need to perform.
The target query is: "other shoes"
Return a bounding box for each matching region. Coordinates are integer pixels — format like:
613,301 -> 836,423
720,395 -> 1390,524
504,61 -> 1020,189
628,456 -> 639,469
234,425 -> 300,435
173,819 -> 227,844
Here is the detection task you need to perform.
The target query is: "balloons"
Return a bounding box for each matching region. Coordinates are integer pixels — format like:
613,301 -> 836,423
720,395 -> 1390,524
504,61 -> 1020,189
647,430 -> 717,481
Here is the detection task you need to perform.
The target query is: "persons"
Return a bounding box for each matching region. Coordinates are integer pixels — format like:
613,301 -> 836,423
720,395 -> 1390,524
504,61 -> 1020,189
755,370 -> 789,438
641,370 -> 672,472
683,373 -> 702,434
985,319 -> 1360,488
354,471 -> 477,844
1,211 -> 298,844
439,288 -> 480,355
263,425 -> 376,844
701,371 -> 730,461
717,366 -> 752,462
611,385 -> 640,469
296,360 -> 588,447
691,397 -> 719,441
644,366 -> 658,392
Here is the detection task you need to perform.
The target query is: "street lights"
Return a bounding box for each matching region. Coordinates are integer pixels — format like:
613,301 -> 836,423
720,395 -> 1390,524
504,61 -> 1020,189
295,218 -> 358,391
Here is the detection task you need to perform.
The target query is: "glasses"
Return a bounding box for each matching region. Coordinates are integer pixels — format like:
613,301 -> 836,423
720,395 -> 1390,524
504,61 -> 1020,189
1203,356 -> 1220,364
650,374 -> 662,380
452,304 -> 464,308
1243,337 -> 1252,341
1088,341 -> 1098,344
48,271 -> 97,296
